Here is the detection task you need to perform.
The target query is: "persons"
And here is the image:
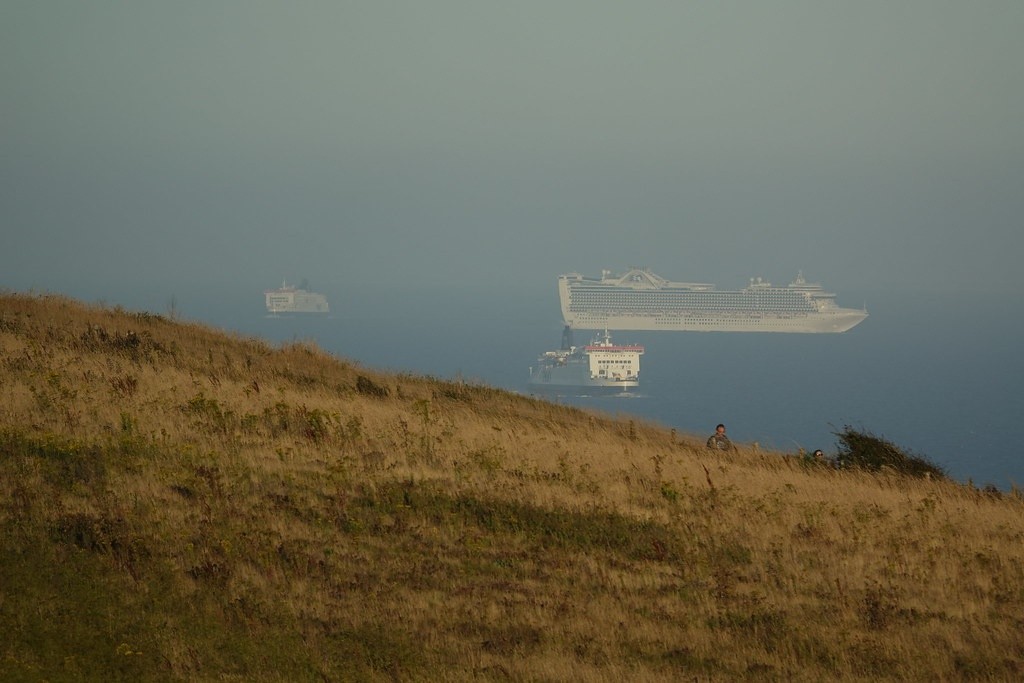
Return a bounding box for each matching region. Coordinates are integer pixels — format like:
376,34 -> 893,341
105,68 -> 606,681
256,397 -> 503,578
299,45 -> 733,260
706,424 -> 733,451
813,450 -> 823,459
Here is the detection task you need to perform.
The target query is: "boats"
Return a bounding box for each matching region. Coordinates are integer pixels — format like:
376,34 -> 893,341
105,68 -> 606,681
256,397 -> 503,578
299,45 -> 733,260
262,278 -> 333,323
528,321 -> 647,391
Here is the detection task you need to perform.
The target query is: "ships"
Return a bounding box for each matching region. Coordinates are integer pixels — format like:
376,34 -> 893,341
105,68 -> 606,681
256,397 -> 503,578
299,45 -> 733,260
556,267 -> 869,335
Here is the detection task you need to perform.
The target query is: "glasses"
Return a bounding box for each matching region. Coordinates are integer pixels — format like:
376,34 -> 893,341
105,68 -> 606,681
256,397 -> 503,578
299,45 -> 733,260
815,453 -> 823,457
